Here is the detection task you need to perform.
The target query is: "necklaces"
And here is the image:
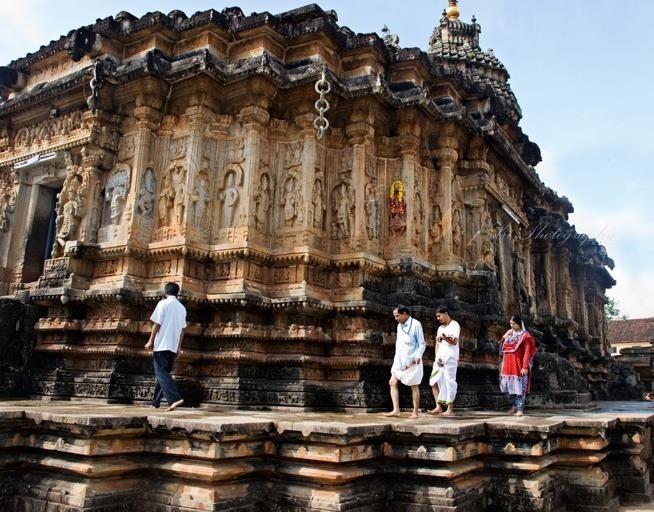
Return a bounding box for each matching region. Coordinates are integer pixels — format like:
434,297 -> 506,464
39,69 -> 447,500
400,319 -> 413,334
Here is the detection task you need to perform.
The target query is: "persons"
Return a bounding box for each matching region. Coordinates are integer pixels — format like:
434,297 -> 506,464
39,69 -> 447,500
426,304 -> 461,417
59,189 -> 85,240
141,281 -> 191,410
498,314 -> 538,416
380,304 -> 426,419
101,163 -> 464,257
